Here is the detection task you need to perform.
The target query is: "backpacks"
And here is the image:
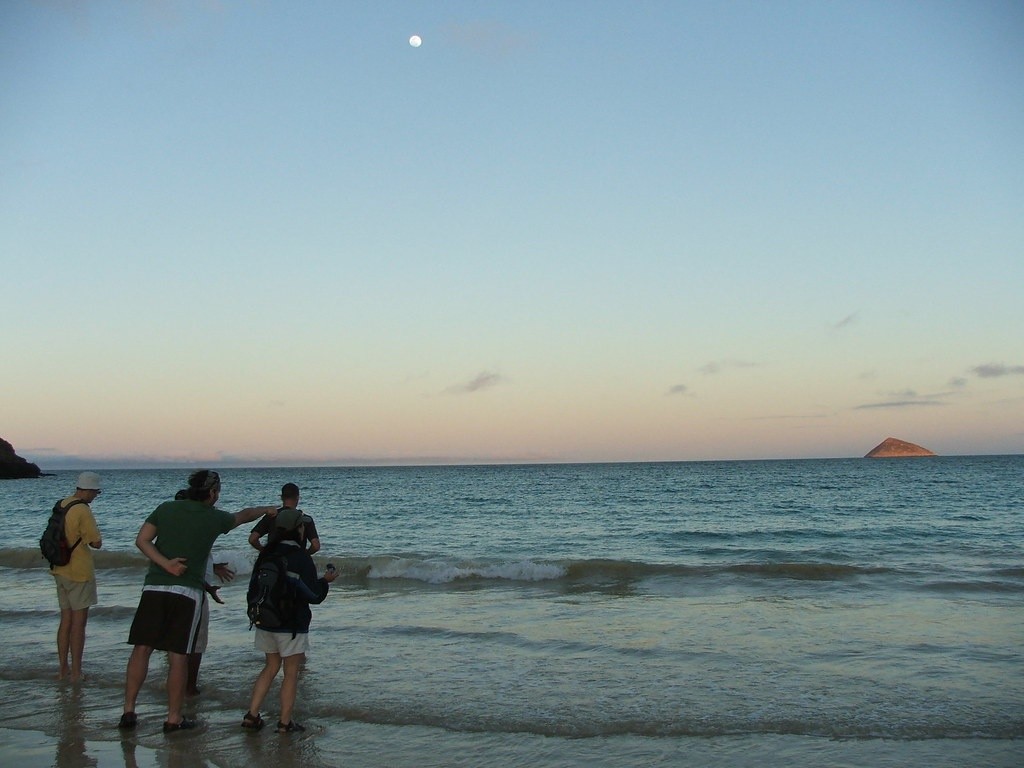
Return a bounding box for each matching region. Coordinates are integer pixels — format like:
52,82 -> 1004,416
39,498 -> 90,570
244,545 -> 307,640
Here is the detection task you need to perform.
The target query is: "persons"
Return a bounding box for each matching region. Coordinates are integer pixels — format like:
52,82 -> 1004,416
114,470 -> 282,732
174,488 -> 235,697
240,509 -> 341,735
248,483 -> 321,557
49,471 -> 103,684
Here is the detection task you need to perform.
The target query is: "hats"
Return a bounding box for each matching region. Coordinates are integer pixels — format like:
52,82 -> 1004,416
77,471 -> 106,490
274,509 -> 311,532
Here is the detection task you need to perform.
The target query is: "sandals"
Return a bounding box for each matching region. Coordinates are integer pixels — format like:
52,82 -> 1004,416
274,719 -> 307,732
118,711 -> 138,728
162,714 -> 206,735
240,711 -> 264,730
